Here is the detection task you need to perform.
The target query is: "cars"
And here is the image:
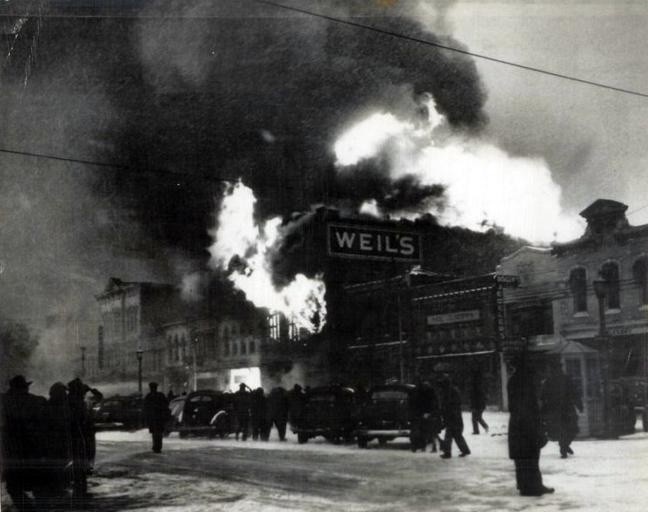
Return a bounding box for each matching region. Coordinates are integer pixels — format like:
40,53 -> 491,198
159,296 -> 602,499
289,384 -> 367,444
608,342 -> 648,432
356,381 -> 441,448
182,385 -> 264,439
161,395 -> 198,439
90,396 -> 148,429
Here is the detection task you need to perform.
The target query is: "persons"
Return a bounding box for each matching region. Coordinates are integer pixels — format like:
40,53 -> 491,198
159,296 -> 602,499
404,353 -> 585,496
1,376 -> 105,512
138,378 -> 308,454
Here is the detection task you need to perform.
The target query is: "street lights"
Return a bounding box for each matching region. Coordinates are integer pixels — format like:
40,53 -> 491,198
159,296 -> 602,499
135,345 -> 144,429
593,269 -> 618,440
79,345 -> 86,382
